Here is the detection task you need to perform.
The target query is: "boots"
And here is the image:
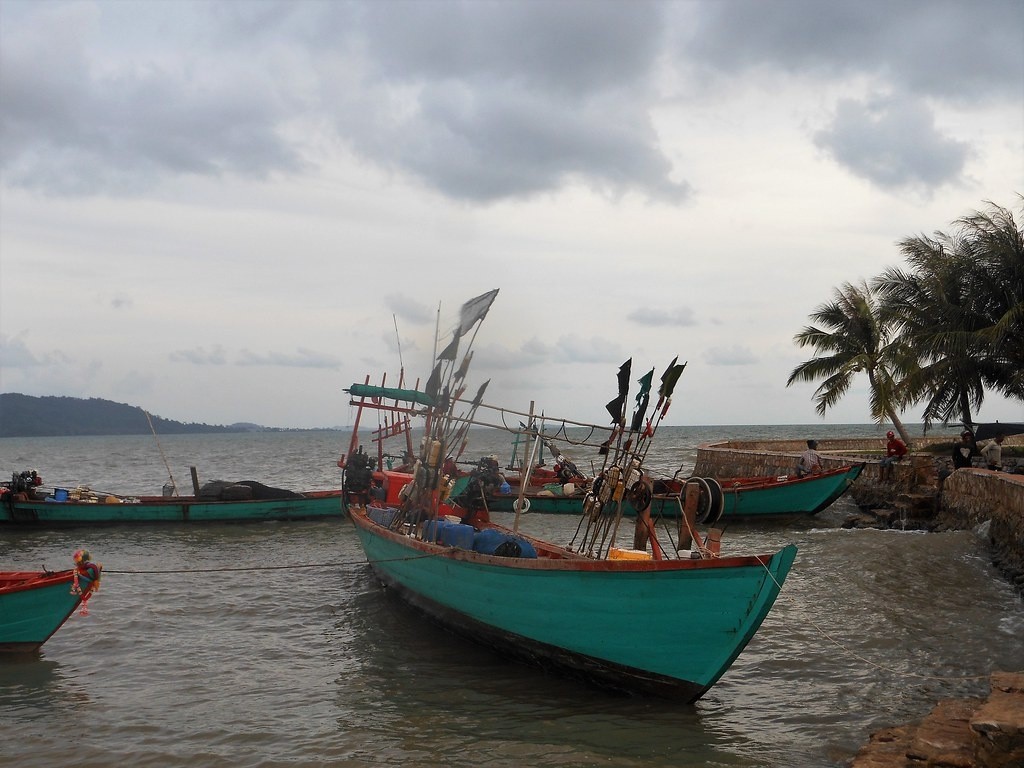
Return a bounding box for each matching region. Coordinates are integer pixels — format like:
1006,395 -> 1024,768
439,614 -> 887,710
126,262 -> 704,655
877,467 -> 884,482
883,466 -> 889,481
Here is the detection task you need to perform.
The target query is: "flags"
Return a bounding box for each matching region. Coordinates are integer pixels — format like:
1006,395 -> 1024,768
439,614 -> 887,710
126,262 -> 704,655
453,288 -> 499,337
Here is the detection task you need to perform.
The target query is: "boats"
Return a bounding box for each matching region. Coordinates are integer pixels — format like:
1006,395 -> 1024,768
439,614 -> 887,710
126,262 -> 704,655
0,464 -> 349,522
370,368 -> 868,523
340,291 -> 801,702
1,565 -> 104,654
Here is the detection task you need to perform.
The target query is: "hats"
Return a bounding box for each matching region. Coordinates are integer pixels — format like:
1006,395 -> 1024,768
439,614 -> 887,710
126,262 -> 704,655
961,430 -> 969,436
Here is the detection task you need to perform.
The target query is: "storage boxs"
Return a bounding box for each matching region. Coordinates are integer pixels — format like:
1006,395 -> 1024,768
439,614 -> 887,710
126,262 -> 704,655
366,505 -> 398,527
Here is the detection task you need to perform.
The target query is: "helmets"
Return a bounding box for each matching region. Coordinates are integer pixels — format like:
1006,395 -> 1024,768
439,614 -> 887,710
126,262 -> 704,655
886,431 -> 894,437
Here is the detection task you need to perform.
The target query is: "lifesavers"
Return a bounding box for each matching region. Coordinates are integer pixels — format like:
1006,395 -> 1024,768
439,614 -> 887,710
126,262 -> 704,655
513,498 -> 530,513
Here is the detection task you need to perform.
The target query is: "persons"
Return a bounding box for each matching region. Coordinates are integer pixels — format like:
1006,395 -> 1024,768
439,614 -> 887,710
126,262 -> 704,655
953,431 -> 976,468
981,433 -> 1004,470
875,431 -> 907,480
796,439 -> 821,477
